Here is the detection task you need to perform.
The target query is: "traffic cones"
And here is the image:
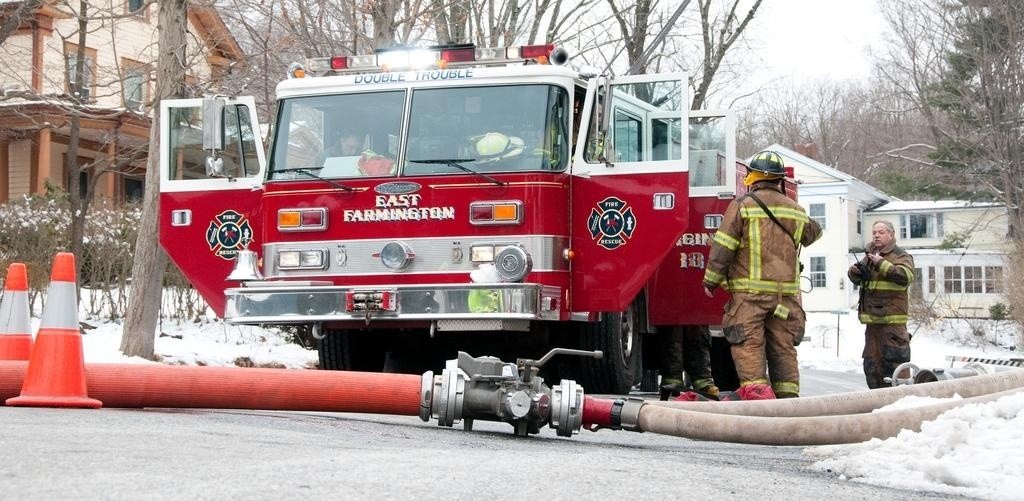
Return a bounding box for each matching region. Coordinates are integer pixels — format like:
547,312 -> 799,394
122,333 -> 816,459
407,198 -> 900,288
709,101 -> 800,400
0,263 -> 33,359
6,253 -> 102,407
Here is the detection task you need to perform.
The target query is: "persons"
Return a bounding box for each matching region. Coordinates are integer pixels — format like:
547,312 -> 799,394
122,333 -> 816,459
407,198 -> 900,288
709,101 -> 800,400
205,151 -> 224,176
701,151 -> 823,400
316,128 -> 364,165
577,100 -> 604,128
848,221 -> 915,390
661,324 -> 720,397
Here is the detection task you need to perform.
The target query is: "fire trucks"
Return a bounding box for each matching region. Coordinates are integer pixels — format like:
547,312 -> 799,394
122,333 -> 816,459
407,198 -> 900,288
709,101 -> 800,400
159,47 -> 812,395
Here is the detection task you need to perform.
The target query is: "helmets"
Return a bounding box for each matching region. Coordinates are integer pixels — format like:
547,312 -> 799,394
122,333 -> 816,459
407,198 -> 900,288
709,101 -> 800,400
474,133 -> 523,164
745,151 -> 786,177
